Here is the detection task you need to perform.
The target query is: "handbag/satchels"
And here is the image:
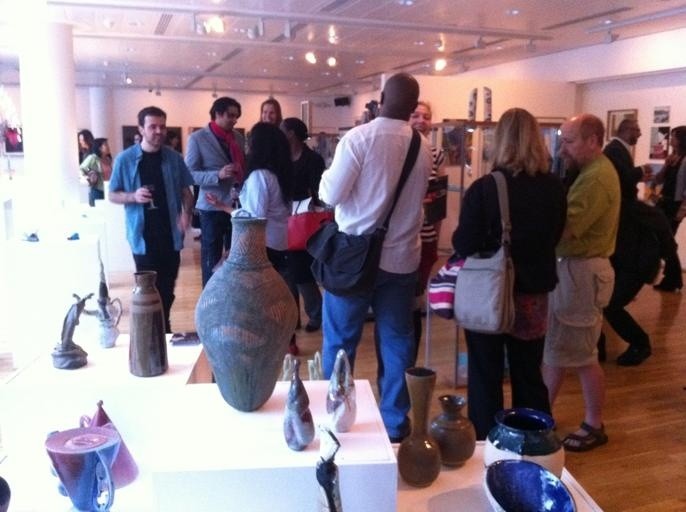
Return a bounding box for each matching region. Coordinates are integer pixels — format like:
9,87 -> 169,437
307,222 -> 385,296
287,211 -> 334,251
429,246 -> 515,333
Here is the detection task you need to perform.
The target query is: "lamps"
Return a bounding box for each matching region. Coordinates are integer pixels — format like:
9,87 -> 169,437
246,15 -> 266,40
283,19 -> 300,40
474,35 -> 487,49
603,28 -> 620,44
523,40 -> 538,53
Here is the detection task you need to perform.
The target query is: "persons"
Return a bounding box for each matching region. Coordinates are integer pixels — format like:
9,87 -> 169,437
79,97 -> 327,354
452,108 -> 567,442
595,118 -> 686,366
541,114 -> 622,452
317,72 -> 434,443
374,102 -> 448,397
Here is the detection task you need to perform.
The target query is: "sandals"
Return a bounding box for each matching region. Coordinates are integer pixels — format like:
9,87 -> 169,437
561,423 -> 608,451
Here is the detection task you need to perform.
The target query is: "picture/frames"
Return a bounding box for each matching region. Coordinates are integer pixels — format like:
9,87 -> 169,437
604,109 -> 638,163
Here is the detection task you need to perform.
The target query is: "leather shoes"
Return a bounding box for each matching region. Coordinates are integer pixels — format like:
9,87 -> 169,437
617,343 -> 651,366
653,275 -> 682,291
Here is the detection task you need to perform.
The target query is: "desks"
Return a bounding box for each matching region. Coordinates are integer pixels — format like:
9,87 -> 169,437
2,332 -> 604,510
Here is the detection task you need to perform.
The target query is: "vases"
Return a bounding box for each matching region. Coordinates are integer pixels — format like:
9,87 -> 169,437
397,368 -> 442,488
192,215 -> 300,411
433,395 -> 478,468
128,270 -> 169,379
